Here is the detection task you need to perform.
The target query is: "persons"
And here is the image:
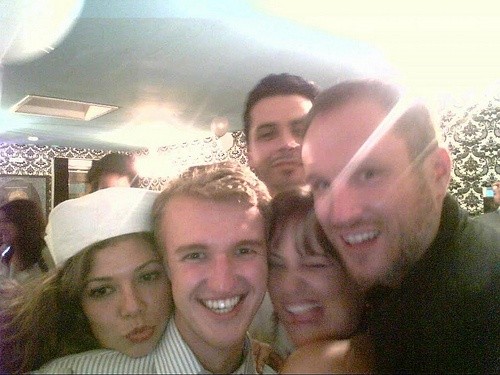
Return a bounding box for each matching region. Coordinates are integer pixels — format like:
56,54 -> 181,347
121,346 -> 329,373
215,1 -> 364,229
0,73 -> 500,375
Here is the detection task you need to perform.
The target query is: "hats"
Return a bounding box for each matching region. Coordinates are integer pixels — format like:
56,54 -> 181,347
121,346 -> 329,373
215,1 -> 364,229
44,187 -> 161,270
